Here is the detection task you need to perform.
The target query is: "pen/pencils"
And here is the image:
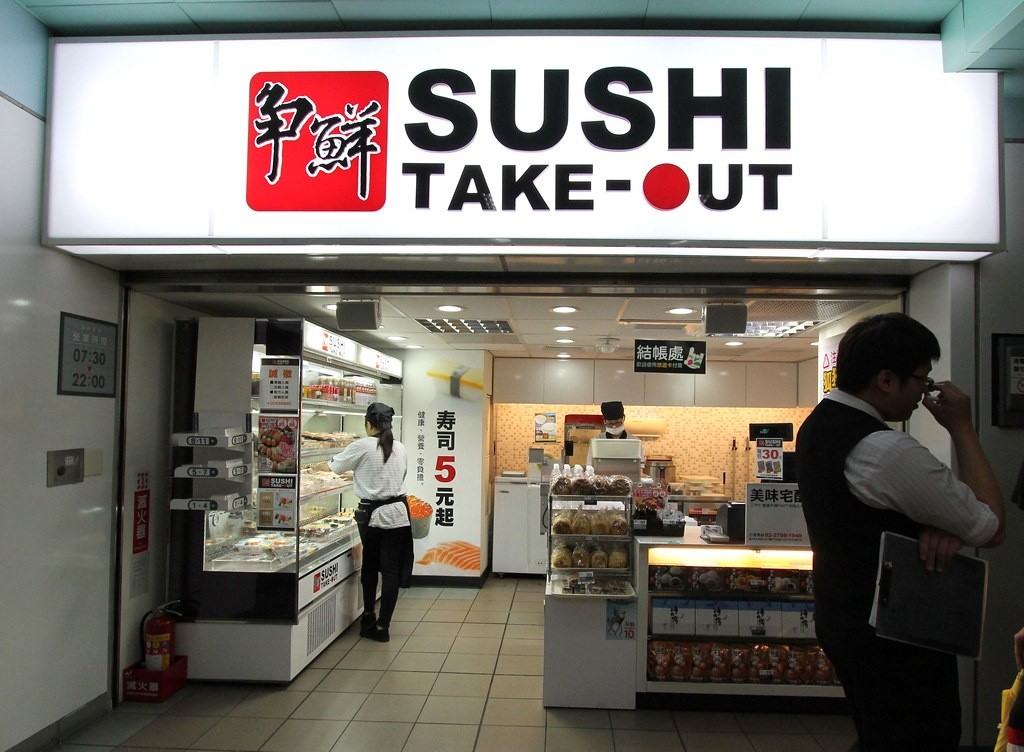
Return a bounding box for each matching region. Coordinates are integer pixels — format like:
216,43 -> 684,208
927,396 -> 944,402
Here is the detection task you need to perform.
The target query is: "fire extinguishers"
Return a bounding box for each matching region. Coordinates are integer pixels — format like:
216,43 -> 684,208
139,599 -> 183,669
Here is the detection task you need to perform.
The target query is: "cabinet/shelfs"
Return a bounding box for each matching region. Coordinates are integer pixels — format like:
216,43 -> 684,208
547,493 -> 634,581
206,394 -> 405,571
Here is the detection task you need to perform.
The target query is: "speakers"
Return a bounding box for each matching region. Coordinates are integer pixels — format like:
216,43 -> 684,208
335,302 -> 382,330
701,304 -> 747,334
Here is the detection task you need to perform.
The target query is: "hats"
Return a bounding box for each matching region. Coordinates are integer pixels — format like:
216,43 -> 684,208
366,402 -> 395,422
601,401 -> 624,420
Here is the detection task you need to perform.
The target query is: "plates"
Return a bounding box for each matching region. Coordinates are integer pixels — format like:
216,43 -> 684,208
535,415 -> 546,424
541,423 -> 557,434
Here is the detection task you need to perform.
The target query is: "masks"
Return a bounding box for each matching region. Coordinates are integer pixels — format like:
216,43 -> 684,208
606,425 -> 625,435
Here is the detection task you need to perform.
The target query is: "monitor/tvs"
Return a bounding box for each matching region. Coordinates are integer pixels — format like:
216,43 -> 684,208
586,439 -> 642,482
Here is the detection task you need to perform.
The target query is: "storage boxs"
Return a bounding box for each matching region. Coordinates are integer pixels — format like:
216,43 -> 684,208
652,597 -> 815,639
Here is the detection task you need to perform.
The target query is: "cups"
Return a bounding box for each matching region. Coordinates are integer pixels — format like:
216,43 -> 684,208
670,483 -> 683,495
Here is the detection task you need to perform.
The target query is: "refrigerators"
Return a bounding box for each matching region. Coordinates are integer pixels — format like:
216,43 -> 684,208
492,472 -> 549,573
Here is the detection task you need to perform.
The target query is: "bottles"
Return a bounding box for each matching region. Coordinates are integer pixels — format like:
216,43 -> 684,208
646,506 -> 656,520
574,464 -> 584,477
633,505 -> 645,520
562,464 -> 572,478
318,377 -> 376,407
550,464 -> 561,493
586,466 -> 595,477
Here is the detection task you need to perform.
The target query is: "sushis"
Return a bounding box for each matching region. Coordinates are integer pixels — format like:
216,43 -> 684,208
301,431 -> 360,449
259,430 -> 296,473
234,508 -> 355,556
300,461 -> 362,496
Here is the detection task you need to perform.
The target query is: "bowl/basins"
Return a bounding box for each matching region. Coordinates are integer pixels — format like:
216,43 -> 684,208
236,534 -> 296,554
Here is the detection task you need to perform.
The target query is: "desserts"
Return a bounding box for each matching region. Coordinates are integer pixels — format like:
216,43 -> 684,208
551,473 -> 632,595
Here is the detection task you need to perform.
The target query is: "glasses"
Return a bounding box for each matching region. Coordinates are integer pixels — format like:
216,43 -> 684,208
605,415 -> 625,428
906,372 -> 935,395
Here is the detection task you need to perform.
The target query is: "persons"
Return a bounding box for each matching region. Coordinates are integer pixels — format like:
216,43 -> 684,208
328,402 -> 415,642
795,312 -> 1007,752
1005,625 -> 1024,752
587,401 -> 645,474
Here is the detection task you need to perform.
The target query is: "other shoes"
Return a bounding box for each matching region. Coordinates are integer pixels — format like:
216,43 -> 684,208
360,612 -> 390,642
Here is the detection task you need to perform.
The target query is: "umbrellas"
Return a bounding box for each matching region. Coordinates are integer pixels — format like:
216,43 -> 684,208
994,668 -> 1024,752
